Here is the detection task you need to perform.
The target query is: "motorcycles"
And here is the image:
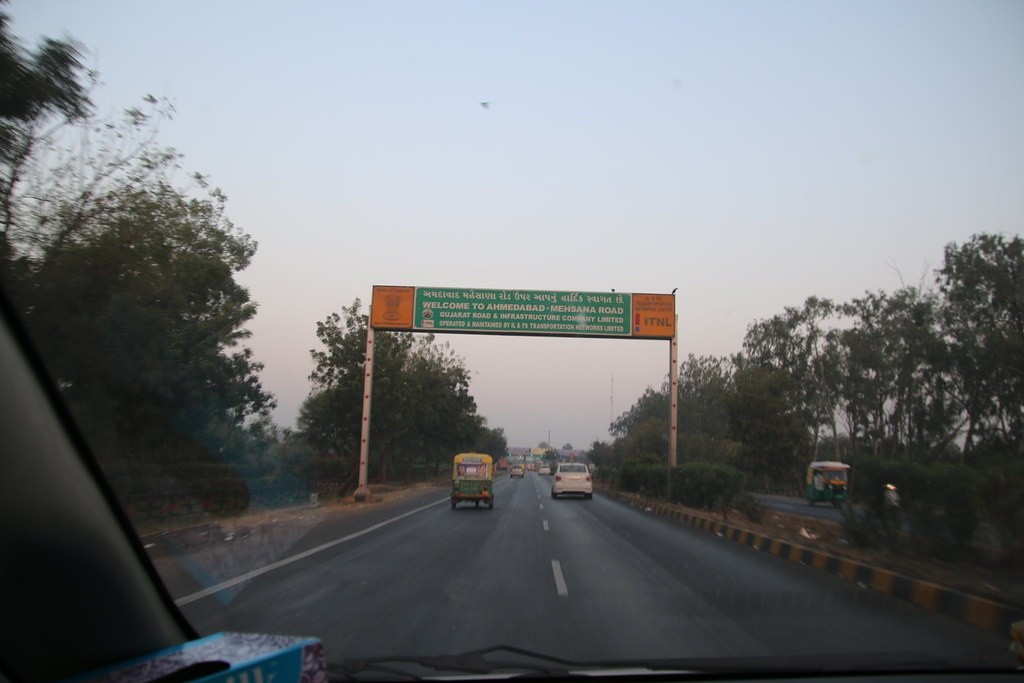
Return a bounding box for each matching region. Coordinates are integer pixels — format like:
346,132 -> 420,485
449,453 -> 495,510
805,460 -> 851,508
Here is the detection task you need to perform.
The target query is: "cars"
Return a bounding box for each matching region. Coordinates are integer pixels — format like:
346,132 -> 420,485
510,465 -> 524,478
539,466 -> 551,476
551,463 -> 593,500
